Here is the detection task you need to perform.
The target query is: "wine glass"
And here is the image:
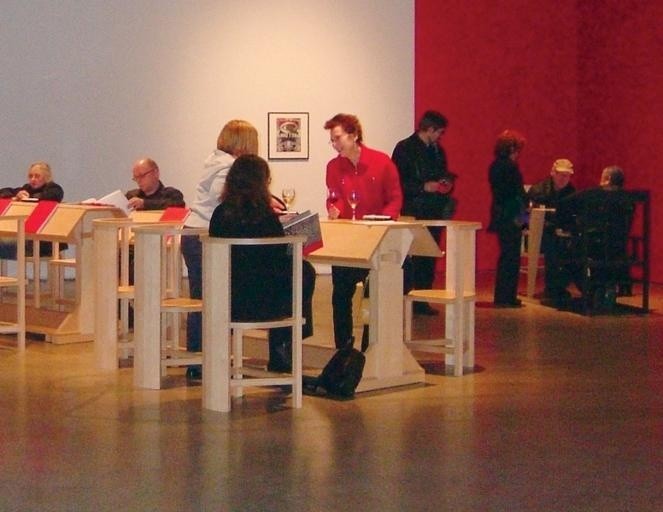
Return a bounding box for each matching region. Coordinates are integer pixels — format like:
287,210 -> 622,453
282,184 -> 295,211
326,187 -> 338,220
347,190 -> 360,220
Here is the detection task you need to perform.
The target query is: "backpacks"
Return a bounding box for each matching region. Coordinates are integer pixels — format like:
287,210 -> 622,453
284,336 -> 366,396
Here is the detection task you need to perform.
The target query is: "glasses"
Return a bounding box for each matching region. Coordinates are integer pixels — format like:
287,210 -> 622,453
132,170 -> 152,181
266,178 -> 272,184
330,132 -> 347,143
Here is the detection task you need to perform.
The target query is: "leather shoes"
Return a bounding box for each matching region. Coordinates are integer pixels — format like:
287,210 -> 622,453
186,367 -> 203,383
413,301 -> 439,315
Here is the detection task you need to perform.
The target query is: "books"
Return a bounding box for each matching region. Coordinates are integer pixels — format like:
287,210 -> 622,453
281,210 -> 325,256
362,214 -> 391,221
157,206 -> 192,245
0,197 -> 13,217
24,199 -> 60,235
81,189 -> 137,217
279,211 -> 299,215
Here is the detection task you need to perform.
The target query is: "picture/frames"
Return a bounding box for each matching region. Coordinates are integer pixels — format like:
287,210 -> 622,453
268,112 -> 309,159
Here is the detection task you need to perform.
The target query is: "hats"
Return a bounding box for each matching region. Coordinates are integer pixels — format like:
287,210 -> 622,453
552,160 -> 574,175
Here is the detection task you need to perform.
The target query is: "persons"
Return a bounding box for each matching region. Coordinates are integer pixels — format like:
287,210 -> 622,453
180,119 -> 285,382
529,157 -> 605,306
0,161 -> 69,257
483,128 -> 532,309
323,112 -> 405,359
209,155 -> 317,376
114,157 -> 185,331
390,111 -> 460,316
553,165 -> 636,315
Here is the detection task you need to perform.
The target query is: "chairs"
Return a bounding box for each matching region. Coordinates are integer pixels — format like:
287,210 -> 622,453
2,215 -> 78,354
515,183 -> 544,306
391,218 -> 483,378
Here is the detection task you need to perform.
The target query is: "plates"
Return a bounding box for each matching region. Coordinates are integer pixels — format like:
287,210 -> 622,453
362,215 -> 390,220
